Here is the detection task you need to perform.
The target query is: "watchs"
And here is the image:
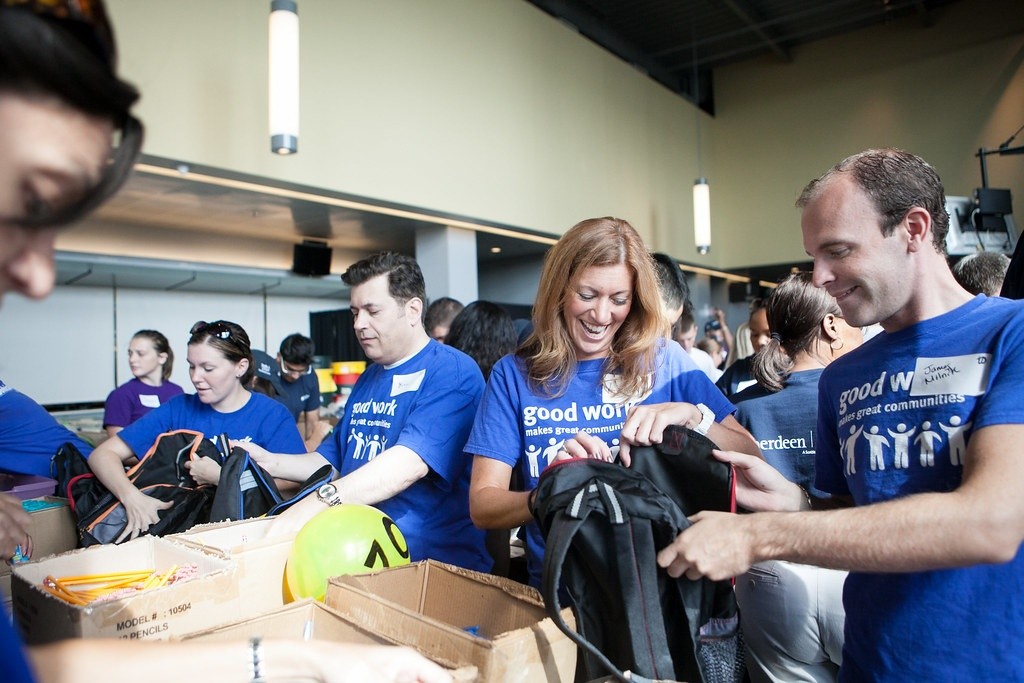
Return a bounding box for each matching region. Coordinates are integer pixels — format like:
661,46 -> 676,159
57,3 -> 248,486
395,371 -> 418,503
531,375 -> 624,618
316,483 -> 342,507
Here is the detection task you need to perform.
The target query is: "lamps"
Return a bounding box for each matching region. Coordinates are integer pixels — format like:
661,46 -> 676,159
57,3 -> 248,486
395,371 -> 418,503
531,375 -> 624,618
689,0 -> 710,256
268,1 -> 298,155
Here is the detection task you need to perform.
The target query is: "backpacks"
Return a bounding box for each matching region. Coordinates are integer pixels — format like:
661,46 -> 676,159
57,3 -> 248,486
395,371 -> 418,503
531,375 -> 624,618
523,424 -> 743,683
76,427 -> 221,547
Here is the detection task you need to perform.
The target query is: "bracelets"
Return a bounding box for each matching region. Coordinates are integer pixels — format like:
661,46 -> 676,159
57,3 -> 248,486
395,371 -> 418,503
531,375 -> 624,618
248,637 -> 265,683
527,487 -> 539,520
797,481 -> 815,511
692,403 -> 715,435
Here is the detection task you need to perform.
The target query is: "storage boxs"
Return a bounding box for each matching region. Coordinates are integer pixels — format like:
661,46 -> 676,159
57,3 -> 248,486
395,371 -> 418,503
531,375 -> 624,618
0,495 -> 577,683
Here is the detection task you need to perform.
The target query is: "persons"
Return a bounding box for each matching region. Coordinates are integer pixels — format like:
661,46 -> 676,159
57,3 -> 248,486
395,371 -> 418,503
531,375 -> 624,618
732,272 -> 868,683
102,330 -> 185,440
424,297 -> 537,384
463,217 -> 766,683
0,491 -> 34,565
0,380 -> 97,482
243,349 -> 333,453
580,230 -> 1023,498
88,320 -> 307,544
0,0 -> 479,683
271,333 -> 319,450
658,148 -> 1024,683
228,254 -> 487,582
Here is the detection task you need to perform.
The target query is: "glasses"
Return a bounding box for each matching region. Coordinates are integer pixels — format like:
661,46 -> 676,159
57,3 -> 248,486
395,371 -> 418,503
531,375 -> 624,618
280,357 -> 312,375
190,321 -> 246,354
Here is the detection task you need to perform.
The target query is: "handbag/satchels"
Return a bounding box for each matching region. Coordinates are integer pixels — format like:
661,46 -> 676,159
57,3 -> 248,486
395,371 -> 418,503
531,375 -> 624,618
48,443 -> 114,522
211,443 -> 336,523
